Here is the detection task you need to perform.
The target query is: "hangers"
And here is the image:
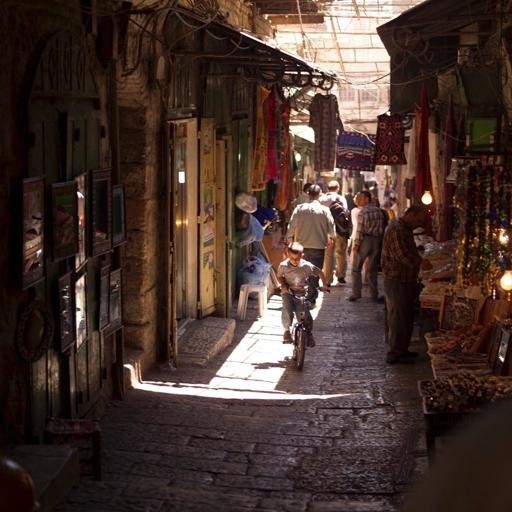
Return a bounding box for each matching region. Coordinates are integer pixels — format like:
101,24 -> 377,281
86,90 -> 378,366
310,87 -> 337,103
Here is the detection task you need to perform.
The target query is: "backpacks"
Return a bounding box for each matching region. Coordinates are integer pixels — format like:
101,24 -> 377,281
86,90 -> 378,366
329,195 -> 353,238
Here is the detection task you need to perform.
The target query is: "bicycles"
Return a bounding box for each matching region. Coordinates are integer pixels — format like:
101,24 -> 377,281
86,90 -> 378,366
280,287 -> 330,371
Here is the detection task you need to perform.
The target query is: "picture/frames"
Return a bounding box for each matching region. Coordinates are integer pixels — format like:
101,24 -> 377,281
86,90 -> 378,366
19,168 -> 127,353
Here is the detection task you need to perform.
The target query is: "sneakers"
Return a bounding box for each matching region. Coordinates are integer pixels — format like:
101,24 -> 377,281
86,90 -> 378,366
385,350 -> 420,365
307,332 -> 316,348
366,294 -> 379,300
345,293 -> 362,302
337,277 -> 346,283
283,329 -> 294,342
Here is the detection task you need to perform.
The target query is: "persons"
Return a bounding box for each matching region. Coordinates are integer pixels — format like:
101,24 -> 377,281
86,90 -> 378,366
0,457 -> 41,512
382,206 -> 433,365
277,242 -> 329,347
284,180 -> 400,301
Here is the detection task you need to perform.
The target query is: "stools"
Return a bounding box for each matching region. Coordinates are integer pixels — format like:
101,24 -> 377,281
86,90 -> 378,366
43,416 -> 103,481
237,285 -> 267,320
